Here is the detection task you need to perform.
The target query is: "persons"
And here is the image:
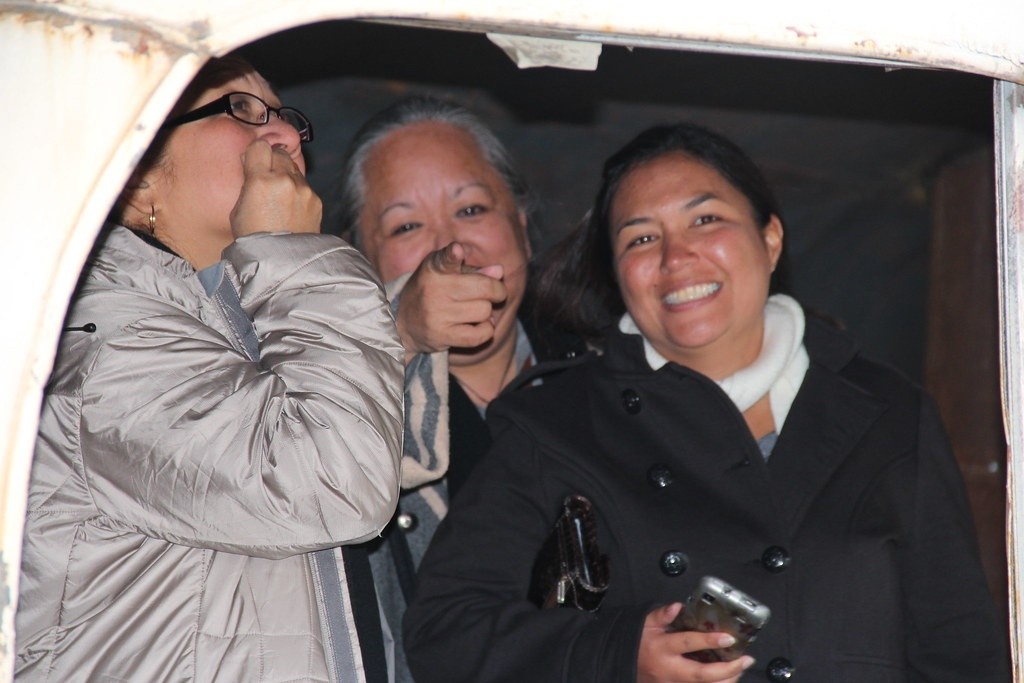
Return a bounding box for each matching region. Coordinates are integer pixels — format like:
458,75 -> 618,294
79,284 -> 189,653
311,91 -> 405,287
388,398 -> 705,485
13,56 -> 405,683
400,119 -> 1013,683
325,87 -> 591,683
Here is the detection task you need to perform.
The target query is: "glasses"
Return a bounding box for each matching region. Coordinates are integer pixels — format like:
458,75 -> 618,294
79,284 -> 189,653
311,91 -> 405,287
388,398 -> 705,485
161,92 -> 313,143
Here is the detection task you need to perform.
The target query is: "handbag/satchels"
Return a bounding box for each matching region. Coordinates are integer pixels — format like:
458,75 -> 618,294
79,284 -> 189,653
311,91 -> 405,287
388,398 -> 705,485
526,495 -> 611,614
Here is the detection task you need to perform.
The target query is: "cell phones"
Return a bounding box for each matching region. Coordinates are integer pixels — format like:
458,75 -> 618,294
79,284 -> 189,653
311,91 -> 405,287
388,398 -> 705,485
666,575 -> 771,665
